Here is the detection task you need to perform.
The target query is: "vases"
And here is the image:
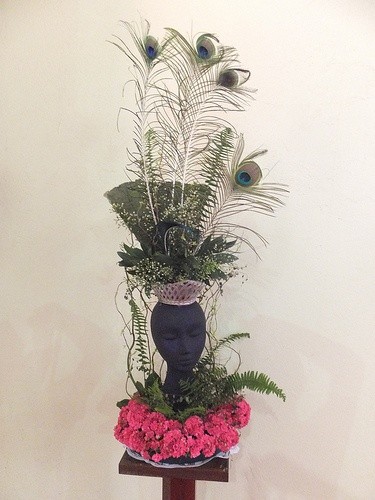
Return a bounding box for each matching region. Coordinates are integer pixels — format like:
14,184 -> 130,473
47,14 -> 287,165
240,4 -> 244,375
124,442 -> 240,468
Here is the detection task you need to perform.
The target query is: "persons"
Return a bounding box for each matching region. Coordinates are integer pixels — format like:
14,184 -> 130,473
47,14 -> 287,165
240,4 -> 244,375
151,301 -> 206,395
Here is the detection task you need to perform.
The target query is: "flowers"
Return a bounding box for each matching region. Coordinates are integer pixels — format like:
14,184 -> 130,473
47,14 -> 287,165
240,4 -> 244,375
94,15 -> 289,464
